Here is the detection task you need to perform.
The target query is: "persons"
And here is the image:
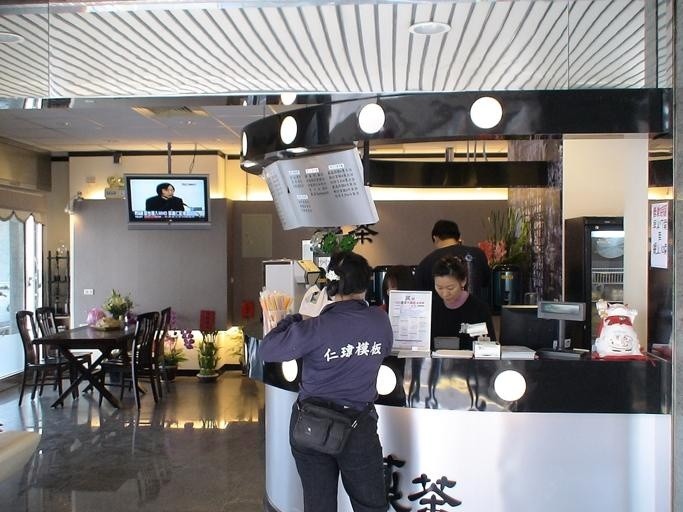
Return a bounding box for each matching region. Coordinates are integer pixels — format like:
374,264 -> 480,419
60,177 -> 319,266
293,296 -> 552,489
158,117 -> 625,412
145,183 -> 184,212
431,256 -> 497,352
378,264 -> 415,313
257,251 -> 394,512
414,219 -> 490,305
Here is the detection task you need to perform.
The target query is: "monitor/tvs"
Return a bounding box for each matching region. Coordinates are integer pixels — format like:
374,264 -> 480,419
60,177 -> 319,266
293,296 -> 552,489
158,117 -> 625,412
124,173 -> 212,229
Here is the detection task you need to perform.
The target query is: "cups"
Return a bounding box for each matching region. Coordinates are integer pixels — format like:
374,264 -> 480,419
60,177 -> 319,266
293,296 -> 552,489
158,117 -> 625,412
262,310 -> 285,338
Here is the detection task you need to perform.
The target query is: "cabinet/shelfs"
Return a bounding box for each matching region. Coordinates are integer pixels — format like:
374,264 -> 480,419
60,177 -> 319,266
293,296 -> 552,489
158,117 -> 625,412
44,248 -> 69,316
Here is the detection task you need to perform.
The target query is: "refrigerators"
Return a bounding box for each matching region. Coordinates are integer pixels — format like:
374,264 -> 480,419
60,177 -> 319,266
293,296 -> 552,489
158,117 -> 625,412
563,216 -> 623,352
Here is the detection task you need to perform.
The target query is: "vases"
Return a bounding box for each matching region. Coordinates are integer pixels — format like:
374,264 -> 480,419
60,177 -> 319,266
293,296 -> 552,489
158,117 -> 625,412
159,364 -> 177,382
196,372 -> 219,382
238,356 -> 247,376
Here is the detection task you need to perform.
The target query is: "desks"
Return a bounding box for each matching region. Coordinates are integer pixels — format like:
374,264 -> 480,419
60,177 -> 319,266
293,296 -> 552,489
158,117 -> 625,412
31,323 -> 154,410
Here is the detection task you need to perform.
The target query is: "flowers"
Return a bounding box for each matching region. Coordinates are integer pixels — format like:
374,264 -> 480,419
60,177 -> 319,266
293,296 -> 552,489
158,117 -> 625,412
476,205 -> 537,267
100,287 -> 137,320
226,301 -> 260,363
196,309 -> 222,374
157,309 -> 194,367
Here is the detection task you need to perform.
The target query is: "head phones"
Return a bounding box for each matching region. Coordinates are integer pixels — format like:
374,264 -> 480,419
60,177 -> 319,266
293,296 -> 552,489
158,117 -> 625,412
327,252 -> 357,296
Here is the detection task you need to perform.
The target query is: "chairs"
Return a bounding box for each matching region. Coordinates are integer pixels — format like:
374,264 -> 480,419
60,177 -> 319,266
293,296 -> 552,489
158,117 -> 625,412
127,305 -> 171,399
15,310 -> 75,408
18,391 -> 176,505
34,307 -> 93,396
96,310 -> 159,408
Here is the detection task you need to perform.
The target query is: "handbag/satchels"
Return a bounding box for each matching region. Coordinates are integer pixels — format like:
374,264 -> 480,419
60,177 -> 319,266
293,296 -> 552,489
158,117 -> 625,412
291,403 -> 353,456
